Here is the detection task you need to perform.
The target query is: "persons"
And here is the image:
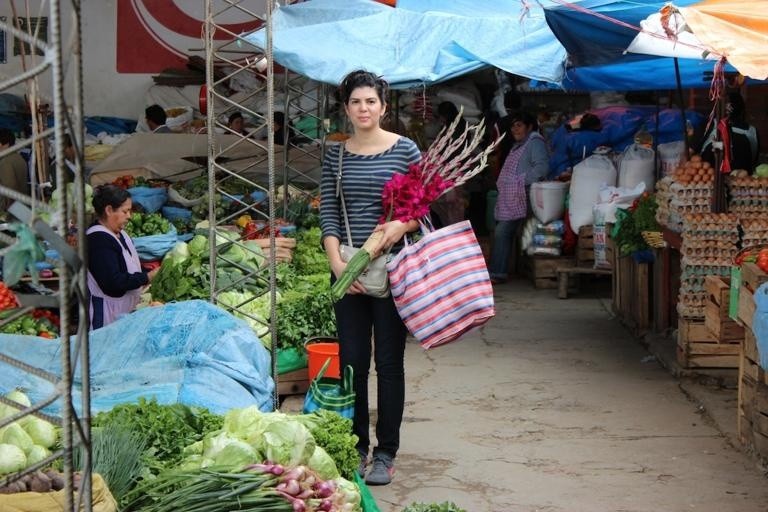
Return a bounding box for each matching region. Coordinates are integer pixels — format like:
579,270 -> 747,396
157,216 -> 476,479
437,100 -> 550,285
318,68 -> 433,487
261,111 -> 298,146
42,134 -> 76,200
222,110 -> 253,139
144,103 -> 177,134
0,126 -> 33,284
68,186 -> 163,330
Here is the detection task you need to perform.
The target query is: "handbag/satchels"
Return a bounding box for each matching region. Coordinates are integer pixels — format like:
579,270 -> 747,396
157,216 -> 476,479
384,214 -> 497,351
339,244 -> 394,299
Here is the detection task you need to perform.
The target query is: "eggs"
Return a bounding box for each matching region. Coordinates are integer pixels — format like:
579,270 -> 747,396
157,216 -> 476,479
656,154 -> 768,313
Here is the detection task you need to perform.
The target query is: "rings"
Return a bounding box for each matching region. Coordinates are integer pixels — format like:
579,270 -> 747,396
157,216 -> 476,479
386,242 -> 392,248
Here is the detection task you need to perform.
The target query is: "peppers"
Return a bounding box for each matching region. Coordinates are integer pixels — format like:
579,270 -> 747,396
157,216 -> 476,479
124,213 -> 169,238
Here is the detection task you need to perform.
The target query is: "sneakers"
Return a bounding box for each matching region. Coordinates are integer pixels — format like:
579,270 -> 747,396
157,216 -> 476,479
365,450 -> 397,487
355,451 -> 369,479
489,273 -> 510,284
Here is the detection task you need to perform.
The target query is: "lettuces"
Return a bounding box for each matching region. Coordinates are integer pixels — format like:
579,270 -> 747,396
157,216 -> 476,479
289,409 -> 361,479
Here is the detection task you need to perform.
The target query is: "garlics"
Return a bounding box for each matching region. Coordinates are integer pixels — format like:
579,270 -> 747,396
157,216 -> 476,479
265,462 -> 354,512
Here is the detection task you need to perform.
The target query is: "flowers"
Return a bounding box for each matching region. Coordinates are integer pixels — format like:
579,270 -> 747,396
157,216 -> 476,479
330,103 -> 508,304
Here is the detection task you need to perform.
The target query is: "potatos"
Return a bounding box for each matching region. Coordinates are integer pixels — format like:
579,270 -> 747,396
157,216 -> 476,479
0,468 -> 81,494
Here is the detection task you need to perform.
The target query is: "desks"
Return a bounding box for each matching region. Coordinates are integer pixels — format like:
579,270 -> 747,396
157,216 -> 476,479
526,255 -> 577,289
574,225 -> 595,266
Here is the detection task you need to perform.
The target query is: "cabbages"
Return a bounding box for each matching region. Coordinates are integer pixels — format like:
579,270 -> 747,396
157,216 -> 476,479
164,231 -> 264,272
181,405 -> 338,482
0,391 -> 57,474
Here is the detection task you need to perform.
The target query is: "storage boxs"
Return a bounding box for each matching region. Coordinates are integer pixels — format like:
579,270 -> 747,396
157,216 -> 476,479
676,261 -> 768,457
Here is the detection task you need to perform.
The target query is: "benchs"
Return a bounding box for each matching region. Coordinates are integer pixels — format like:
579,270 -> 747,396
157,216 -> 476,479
555,264 -> 613,300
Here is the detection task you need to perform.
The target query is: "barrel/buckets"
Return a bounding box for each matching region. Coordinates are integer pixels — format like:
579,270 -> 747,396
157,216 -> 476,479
304,337 -> 341,384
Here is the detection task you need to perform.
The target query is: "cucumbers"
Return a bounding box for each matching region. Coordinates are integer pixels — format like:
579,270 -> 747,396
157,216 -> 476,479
199,242 -> 269,293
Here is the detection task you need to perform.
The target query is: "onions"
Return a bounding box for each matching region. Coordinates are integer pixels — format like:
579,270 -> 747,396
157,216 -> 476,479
40,269 -> 52,278
